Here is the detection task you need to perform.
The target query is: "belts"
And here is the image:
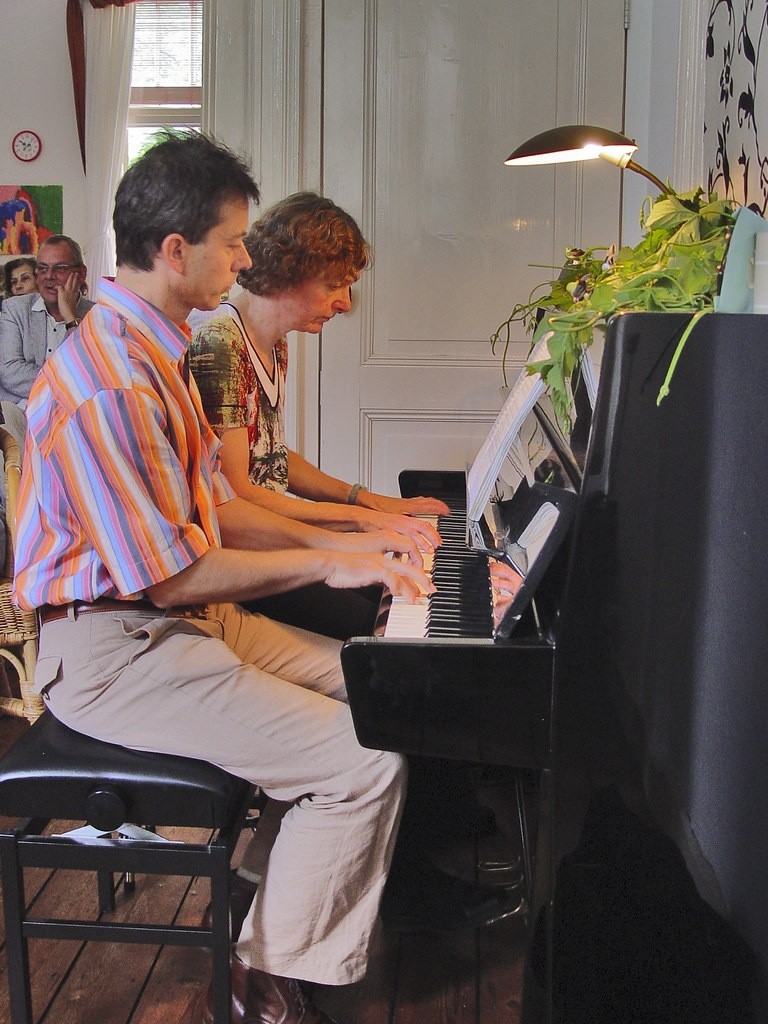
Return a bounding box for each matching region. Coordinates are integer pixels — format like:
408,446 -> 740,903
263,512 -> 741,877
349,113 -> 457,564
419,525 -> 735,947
41,597 -> 191,626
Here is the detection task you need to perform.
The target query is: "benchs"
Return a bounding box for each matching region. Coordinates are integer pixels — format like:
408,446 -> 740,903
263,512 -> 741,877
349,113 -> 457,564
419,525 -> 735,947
0,711 -> 269,1024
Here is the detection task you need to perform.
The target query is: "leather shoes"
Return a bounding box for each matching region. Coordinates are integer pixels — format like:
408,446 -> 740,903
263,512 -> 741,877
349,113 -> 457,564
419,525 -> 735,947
199,868 -> 262,938
202,941 -> 338,1024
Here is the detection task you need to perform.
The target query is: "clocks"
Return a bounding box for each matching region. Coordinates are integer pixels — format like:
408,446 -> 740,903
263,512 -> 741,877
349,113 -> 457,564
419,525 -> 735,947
12,130 -> 42,162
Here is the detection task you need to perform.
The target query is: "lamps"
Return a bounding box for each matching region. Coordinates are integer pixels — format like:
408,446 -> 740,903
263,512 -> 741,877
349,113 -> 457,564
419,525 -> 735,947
505,124 -> 675,196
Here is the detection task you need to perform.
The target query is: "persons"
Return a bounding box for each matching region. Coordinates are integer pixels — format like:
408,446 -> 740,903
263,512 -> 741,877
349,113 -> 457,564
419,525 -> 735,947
488,562 -> 525,607
0,234 -> 98,412
11,125 -> 434,1024
4,258 -> 38,297
185,190 -> 449,636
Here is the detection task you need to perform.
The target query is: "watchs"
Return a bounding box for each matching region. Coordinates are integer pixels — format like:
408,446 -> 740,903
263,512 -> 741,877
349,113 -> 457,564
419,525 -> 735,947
348,482 -> 368,506
66,318 -> 82,331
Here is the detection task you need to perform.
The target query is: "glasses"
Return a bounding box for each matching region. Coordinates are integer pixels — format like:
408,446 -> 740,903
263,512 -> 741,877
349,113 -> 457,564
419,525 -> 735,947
35,264 -> 83,276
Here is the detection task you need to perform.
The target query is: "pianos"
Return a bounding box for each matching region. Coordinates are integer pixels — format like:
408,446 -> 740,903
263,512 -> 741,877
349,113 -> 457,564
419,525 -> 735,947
340,470 -> 589,974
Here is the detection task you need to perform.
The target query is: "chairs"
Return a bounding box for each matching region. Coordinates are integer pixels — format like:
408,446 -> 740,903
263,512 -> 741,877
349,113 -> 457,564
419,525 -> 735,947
0,399 -> 46,725
